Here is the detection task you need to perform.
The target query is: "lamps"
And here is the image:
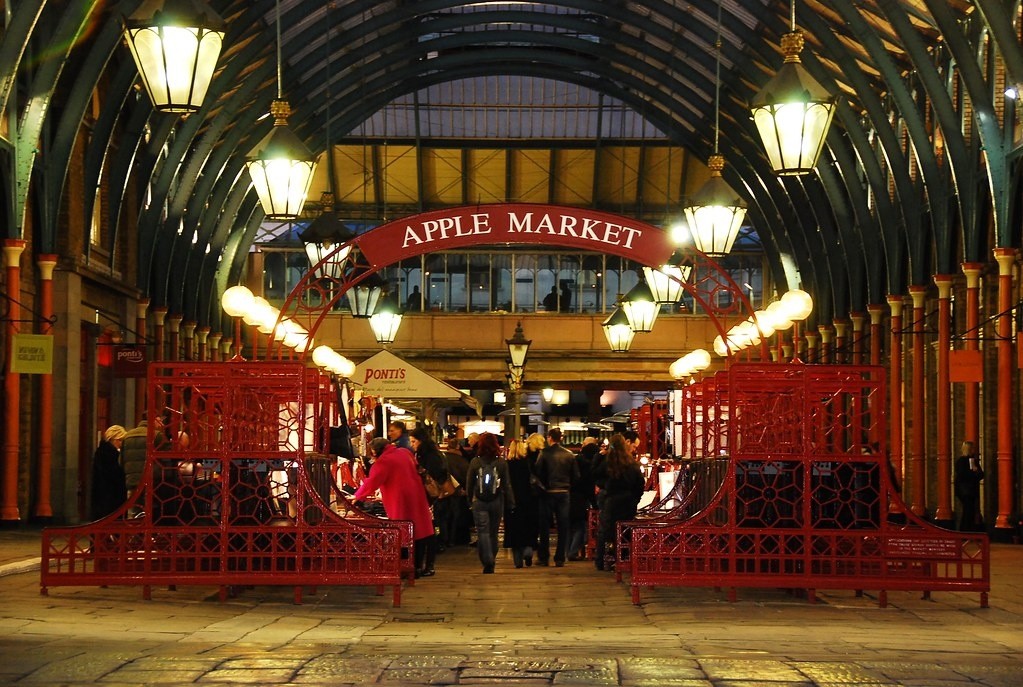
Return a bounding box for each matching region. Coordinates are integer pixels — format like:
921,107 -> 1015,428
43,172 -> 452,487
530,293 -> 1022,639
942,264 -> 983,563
670,288 -> 814,383
603,300 -> 636,353
118,0 -> 226,113
298,0 -> 354,280
642,0 -> 693,303
747,0 -> 840,176
243,0 -> 319,220
682,0 -> 748,256
345,67 -> 382,318
369,183 -> 404,345
617,270 -> 663,334
220,286 -> 358,385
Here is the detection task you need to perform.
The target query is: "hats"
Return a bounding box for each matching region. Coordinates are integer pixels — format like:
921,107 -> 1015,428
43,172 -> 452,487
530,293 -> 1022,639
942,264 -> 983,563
105,425 -> 128,442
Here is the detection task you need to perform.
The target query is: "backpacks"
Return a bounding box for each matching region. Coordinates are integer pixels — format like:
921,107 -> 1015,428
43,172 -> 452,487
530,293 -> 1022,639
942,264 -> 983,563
475,456 -> 503,502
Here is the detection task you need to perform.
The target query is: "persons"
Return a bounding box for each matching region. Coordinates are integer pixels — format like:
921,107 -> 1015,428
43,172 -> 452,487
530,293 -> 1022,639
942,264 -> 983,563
542,286 -> 562,310
503,427 -> 582,567
346,437 -> 435,580
953,441 -> 985,534
566,430 -> 645,572
405,285 -> 426,311
467,431 -> 518,575
560,280 -> 572,313
861,443 -> 902,495
386,421 -> 480,554
87,407 -> 192,557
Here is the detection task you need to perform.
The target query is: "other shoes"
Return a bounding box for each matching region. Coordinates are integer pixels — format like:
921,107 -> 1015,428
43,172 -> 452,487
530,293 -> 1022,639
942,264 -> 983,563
516,563 -> 523,568
595,558 -> 605,571
556,561 -> 565,567
524,552 -> 532,567
415,568 -> 423,579
483,557 -> 495,574
534,559 -> 548,566
422,568 -> 435,576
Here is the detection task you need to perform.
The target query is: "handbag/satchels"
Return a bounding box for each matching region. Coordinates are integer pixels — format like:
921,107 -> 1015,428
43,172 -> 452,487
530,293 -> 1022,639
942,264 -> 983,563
319,424 -> 355,461
424,474 -> 455,499
